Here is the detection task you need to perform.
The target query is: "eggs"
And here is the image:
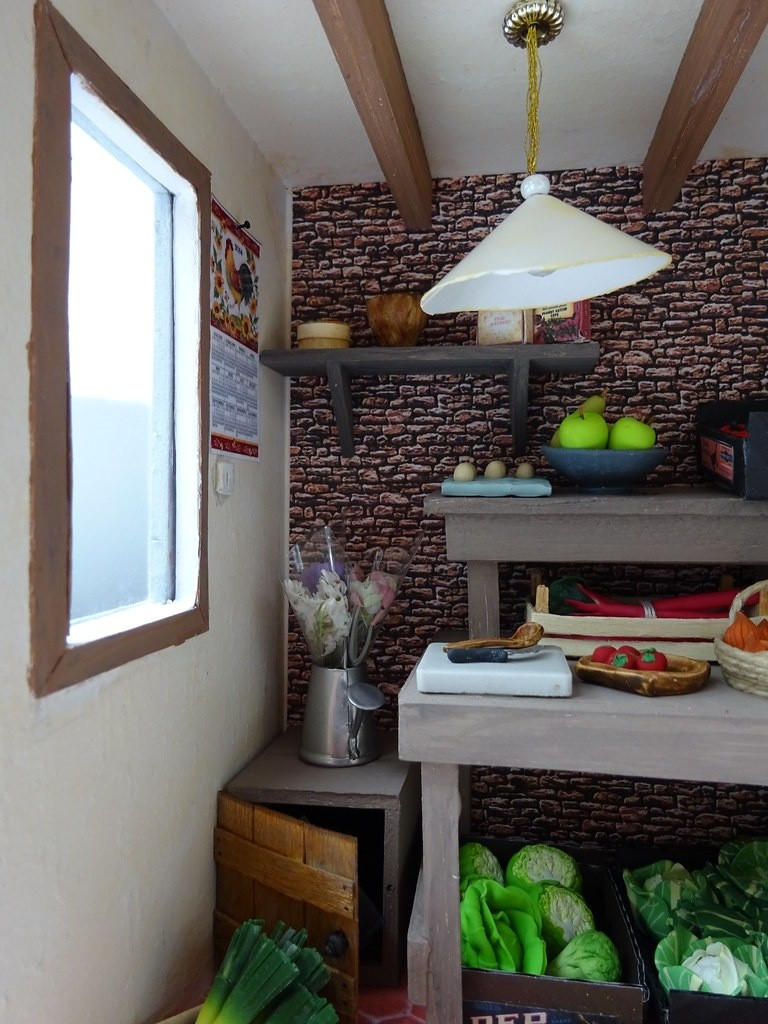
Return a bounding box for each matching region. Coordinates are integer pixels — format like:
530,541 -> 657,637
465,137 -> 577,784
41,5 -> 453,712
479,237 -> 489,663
453,460 -> 533,481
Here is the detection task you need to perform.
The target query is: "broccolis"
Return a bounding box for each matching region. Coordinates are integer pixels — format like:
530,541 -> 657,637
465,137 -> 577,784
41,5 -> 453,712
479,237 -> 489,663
622,835 -> 768,999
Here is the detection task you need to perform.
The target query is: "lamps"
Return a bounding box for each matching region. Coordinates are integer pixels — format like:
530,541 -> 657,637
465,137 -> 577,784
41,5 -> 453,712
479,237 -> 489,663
422,0 -> 669,319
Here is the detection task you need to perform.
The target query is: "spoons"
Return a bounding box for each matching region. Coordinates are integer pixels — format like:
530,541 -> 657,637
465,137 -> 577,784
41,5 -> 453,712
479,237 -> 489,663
442,621 -> 543,653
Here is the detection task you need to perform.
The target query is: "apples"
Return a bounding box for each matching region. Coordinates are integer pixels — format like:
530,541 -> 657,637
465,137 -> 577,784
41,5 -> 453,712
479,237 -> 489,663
560,407 -> 658,450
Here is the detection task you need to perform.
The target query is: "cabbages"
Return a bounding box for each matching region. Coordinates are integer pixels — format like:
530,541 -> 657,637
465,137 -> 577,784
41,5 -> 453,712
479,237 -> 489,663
549,576 -> 589,614
460,839 -> 619,980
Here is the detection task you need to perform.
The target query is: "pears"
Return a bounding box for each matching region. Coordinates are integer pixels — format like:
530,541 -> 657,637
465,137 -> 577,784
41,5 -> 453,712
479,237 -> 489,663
551,388 -> 610,448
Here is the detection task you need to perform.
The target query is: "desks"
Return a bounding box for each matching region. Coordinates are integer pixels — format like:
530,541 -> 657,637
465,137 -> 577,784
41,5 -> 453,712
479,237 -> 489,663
396,656 -> 768,1024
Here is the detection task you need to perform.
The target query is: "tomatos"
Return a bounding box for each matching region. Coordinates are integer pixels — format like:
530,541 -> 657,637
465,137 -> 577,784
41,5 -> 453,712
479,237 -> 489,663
593,644 -> 666,672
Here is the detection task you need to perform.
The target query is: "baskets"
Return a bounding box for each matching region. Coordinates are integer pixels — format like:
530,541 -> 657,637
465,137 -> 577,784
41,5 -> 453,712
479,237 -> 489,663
712,579 -> 768,700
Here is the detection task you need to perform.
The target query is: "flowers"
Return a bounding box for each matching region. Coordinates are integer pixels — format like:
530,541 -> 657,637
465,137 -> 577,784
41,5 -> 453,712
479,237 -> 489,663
348,567 -> 397,665
282,560 -> 348,667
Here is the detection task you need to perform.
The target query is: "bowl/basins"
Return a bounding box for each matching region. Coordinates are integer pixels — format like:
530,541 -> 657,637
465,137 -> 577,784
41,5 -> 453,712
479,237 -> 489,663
363,292 -> 426,347
538,442 -> 670,491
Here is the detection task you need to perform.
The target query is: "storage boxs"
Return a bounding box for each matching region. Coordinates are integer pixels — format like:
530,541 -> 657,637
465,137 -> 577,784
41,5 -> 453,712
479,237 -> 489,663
452,845 -> 646,1024
607,862 -> 768,1024
526,568 -> 766,662
714,579 -> 766,697
695,400 -> 768,499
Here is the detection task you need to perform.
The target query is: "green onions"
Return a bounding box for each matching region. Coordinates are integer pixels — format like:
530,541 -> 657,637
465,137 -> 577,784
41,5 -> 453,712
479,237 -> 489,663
194,917 -> 340,1024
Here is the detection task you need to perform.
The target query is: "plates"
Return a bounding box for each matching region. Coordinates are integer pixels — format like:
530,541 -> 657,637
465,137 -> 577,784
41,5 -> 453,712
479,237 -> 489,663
575,649 -> 712,697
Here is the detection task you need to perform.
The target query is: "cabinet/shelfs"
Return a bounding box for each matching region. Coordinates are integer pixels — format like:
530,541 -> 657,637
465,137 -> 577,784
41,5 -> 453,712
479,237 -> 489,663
259,345 -> 600,454
210,731 -> 422,1018
426,482 -> 768,641
